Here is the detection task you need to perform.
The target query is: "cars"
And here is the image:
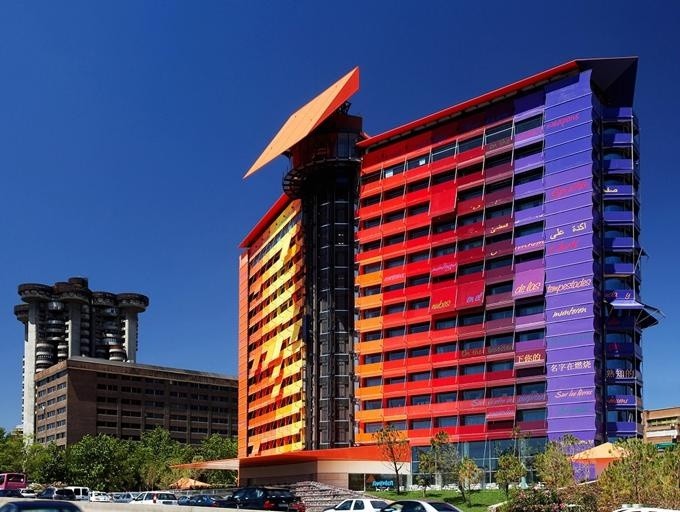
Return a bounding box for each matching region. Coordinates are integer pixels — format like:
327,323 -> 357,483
0,500 -> 82,512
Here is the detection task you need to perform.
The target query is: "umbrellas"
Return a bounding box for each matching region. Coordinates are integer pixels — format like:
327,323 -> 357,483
566,441 -> 630,463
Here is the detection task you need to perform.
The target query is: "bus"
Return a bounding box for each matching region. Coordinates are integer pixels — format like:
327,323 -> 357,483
0,473 -> 27,497
